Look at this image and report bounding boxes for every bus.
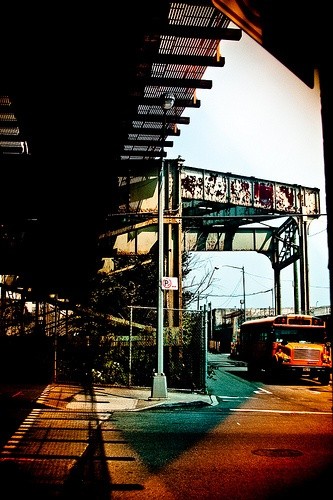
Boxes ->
[235,313,332,385]
